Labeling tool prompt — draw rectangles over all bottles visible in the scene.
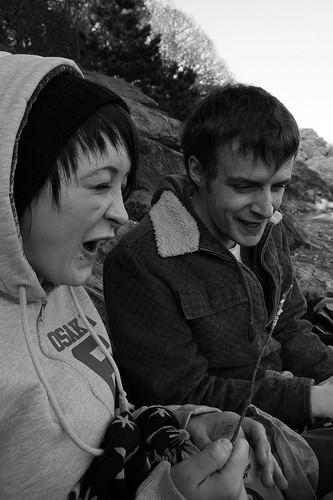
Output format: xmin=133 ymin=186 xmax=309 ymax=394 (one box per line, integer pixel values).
xmin=209 ymin=422 xmax=286 ymax=500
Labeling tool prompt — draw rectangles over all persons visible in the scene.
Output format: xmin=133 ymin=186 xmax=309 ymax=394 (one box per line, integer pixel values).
xmin=103 ymin=84 xmax=333 ymax=500
xmin=0 ymin=53 xmax=286 ymax=500
